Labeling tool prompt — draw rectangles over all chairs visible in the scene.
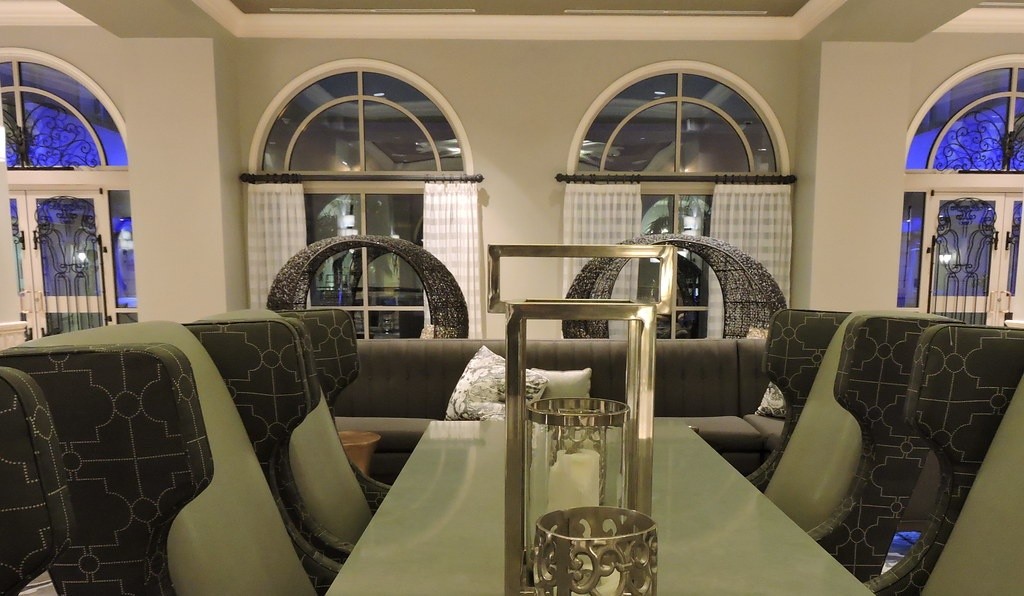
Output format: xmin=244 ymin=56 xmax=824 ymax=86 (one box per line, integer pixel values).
xmin=0 ymin=318 xmax=344 ymax=596
xmin=180 ymin=307 xmax=394 ymax=562
xmin=0 ymin=367 xmax=75 ymax=596
xmin=739 ymin=339 xmax=787 ymax=467
xmin=862 ymin=325 xmax=1024 ymax=596
xmin=745 ymin=308 xmax=966 ymax=583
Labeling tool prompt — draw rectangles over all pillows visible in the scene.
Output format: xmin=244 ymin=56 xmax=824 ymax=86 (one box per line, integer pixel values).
xmin=442 ymin=345 xmax=550 ymax=420
xmin=755 ymin=381 xmax=786 ymax=420
xmin=530 ymin=368 xmax=592 ymax=398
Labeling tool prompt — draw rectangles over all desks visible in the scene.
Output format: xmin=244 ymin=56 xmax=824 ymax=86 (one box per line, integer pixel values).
xmin=324 ymin=421 xmax=877 ymax=596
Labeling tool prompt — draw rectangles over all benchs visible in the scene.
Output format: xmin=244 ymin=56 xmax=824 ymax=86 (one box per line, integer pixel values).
xmin=332 ymin=339 xmax=763 ymax=471
